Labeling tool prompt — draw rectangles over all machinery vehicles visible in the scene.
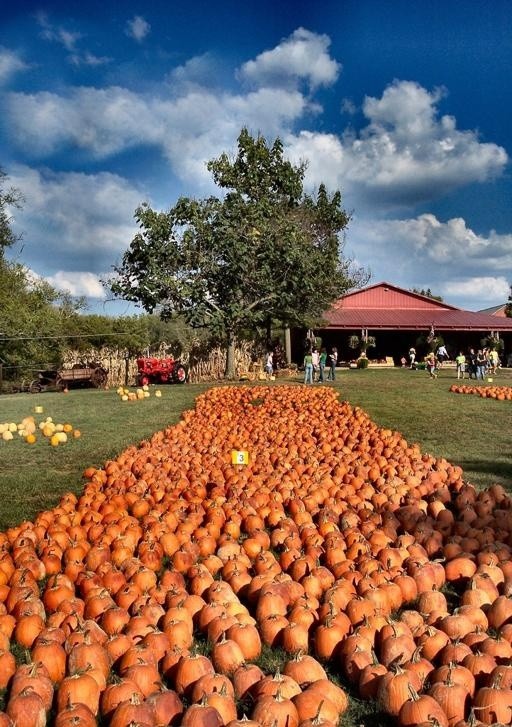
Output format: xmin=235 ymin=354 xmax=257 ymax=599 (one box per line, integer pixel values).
xmin=131 ymin=354 xmax=192 ymax=385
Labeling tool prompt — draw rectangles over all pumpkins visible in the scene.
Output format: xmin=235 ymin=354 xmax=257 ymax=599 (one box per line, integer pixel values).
xmin=0 ymin=384 xmax=512 ymax=727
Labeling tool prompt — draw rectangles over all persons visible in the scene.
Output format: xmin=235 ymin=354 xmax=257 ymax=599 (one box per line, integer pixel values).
xmin=357 ymin=352 xmax=369 ymax=361
xmin=265 ymin=351 xmax=274 ymax=376
xmin=63 ymin=354 xmax=90 ymax=369
xmin=298 ymin=347 xmax=338 ymax=385
xmin=400 ymin=333 xmax=505 ymax=382
